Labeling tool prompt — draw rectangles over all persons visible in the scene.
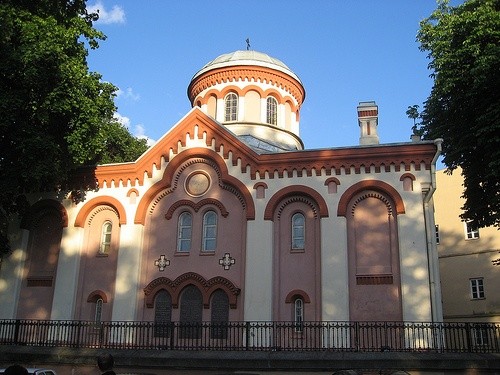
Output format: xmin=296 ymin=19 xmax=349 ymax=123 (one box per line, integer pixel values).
xmin=98 ymin=352 xmax=116 ymax=375
xmin=4 ymin=365 xmax=28 ymax=375
